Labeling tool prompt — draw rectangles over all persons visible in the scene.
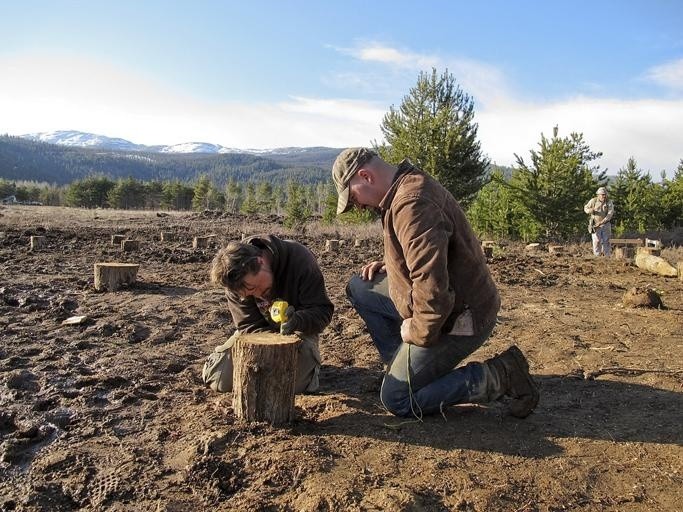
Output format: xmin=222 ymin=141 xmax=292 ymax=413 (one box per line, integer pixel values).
xmin=582 ymin=186 xmax=614 ymax=257
xmin=200 ymin=235 xmax=333 ymax=399
xmin=331 ymin=148 xmax=538 ymax=417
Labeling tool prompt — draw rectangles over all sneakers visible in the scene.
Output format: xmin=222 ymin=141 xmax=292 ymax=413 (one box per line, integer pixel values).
xmin=493 ymin=345 xmax=540 ymax=419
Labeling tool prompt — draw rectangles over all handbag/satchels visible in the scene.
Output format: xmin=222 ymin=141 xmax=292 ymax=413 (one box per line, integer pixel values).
xmin=587 ymin=218 xmax=597 ymax=234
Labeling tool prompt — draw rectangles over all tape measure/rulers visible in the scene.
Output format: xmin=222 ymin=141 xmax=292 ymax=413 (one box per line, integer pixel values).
xmin=269 ymin=300 xmax=288 ymax=336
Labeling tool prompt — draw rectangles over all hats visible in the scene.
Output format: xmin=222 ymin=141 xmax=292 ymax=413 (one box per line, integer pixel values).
xmin=596 ymin=187 xmax=608 ymax=195
xmin=331 ymin=145 xmax=379 ymax=216
